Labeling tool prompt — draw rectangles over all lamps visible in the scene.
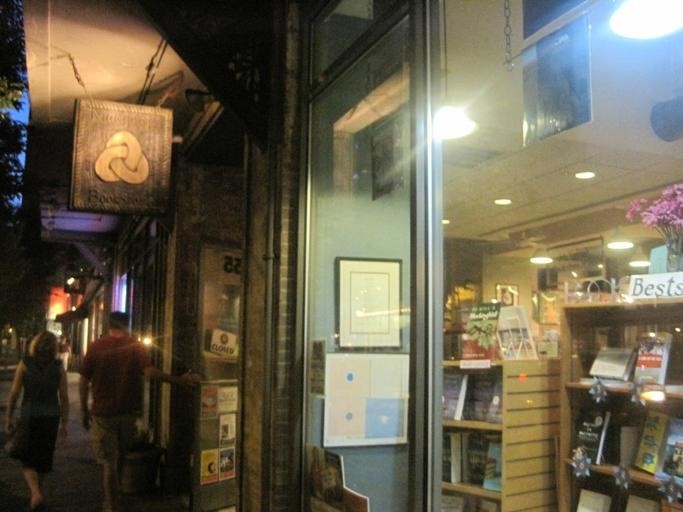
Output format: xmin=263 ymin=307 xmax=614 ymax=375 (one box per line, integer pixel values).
xmin=182 ymin=86 xmax=216 ymax=115
xmin=628 ymin=244 xmax=652 ymax=269
xmin=607 ymin=229 xmax=636 ymax=251
xmin=528 ymin=244 xmax=554 ymax=265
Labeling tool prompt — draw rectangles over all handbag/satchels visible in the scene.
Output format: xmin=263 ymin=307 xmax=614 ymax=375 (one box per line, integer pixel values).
xmin=2 ymin=422 xmax=32 ymax=460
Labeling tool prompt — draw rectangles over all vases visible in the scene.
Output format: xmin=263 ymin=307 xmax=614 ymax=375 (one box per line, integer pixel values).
xmin=663 ymin=239 xmax=682 ymax=274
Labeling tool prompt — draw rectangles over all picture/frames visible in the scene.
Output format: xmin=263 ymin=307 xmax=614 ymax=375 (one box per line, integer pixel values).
xmin=494 ymin=281 xmax=519 ymax=309
xmin=333 ymin=255 xmax=403 ymax=352
xmin=319 ymin=354 xmax=411 ymax=447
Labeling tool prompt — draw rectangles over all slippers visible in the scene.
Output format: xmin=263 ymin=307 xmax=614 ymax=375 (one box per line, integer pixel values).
xmin=20 ymin=496 xmax=46 ymax=512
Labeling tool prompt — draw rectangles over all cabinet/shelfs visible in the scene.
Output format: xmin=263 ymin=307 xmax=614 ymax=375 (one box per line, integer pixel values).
xmin=442 ymin=357 xmax=557 ymax=509
xmin=557 ymin=296 xmax=683 ymax=509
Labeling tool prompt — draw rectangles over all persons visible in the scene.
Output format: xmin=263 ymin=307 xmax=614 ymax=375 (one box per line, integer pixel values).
xmin=3 ymin=328 xmax=71 ymax=510
xmin=77 ymin=309 xmax=202 ymax=511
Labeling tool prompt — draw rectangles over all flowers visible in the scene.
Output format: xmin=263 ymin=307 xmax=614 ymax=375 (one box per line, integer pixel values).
xmin=623 ymin=176 xmax=683 ymax=240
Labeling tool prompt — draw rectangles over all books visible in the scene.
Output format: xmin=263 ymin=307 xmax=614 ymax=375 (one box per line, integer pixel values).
xmin=442 ymin=372 xmax=503 ymax=424
xmin=576 ymin=489 xmax=683 ymax=512
xmin=571 ymin=406 xmax=683 ymax=480
xmin=443 ymin=301 xmax=537 ymax=360
xmin=440 ymin=491 xmax=499 ymax=512
xmin=442 ymin=428 xmax=502 ymax=493
xmin=588 ymin=331 xmax=673 ymax=385
